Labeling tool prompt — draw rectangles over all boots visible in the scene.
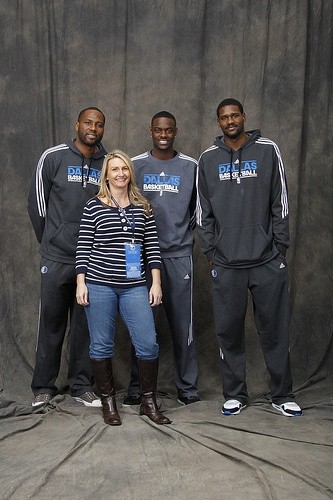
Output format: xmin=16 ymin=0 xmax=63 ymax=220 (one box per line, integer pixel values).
xmin=90 ymin=358 xmax=122 ymax=425
xmin=135 ymin=354 xmax=171 ymax=423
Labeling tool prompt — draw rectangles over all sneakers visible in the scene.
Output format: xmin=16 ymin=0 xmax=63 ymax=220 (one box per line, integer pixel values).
xmin=272 ymin=400 xmax=303 ymax=417
xmin=71 ymin=391 xmax=102 ymax=408
xmin=221 ymin=399 xmax=247 ymax=416
xmin=121 ymin=393 xmax=143 ymax=408
xmin=176 ymin=394 xmax=201 ymax=407
xmin=31 ymin=393 xmax=52 ymax=406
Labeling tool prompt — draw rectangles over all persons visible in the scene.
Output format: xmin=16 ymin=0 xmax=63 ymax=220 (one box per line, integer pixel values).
xmin=27 ymin=108 xmax=108 ymax=407
xmin=74 ymin=150 xmax=171 ymax=425
xmin=122 ymin=111 xmax=198 ymax=407
xmin=195 ymin=98 xmax=303 ymax=417
